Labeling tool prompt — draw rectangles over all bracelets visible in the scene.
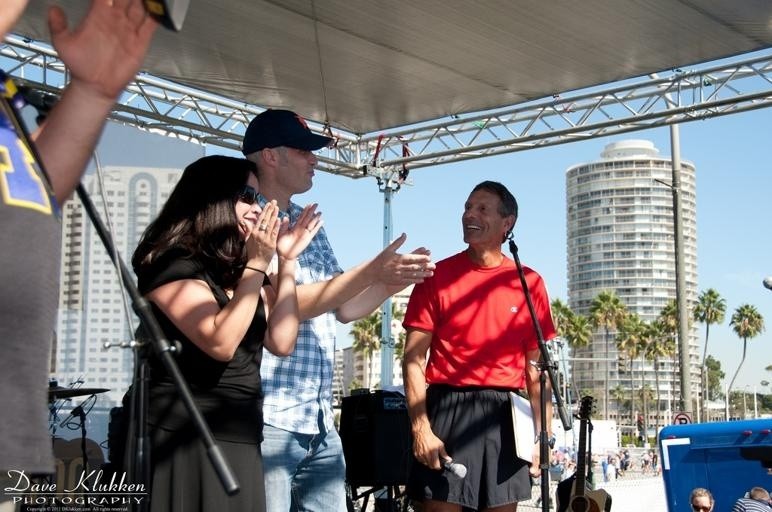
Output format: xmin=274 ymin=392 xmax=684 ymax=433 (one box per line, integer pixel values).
xmin=245 ymin=266 xmax=266 ymax=276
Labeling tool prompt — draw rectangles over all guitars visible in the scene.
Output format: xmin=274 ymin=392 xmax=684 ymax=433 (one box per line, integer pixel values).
xmin=555 ymin=395 xmax=612 ymax=512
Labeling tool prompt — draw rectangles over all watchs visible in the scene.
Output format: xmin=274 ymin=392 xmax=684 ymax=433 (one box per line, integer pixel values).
xmin=534 ymin=433 xmax=556 ymax=449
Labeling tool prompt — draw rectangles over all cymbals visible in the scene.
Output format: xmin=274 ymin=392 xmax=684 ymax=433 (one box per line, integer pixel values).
xmin=48 ymin=385 xmax=110 ymax=403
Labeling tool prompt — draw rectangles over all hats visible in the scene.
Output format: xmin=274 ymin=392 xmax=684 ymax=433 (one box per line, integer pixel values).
xmin=241 ymin=108 xmax=332 ymax=156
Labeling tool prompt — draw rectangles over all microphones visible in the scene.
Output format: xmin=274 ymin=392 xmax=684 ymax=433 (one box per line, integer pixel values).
xmin=762 ymin=277 xmax=772 ymax=291
xmin=438 ymin=456 xmax=468 ymax=480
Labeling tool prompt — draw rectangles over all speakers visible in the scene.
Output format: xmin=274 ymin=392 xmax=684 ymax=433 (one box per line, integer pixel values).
xmin=340 ymin=409 xmax=409 ymax=484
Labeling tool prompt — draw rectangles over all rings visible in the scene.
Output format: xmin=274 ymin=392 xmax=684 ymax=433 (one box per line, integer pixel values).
xmin=260 ymin=224 xmax=266 ymax=230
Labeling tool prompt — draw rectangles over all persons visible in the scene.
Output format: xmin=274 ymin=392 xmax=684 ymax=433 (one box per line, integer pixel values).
xmin=534 ymin=448 xmax=658 ymax=508
xmin=402 ymin=181 xmax=557 ymax=512
xmin=123 ymin=154 xmax=324 ymax=512
xmin=690 ymin=488 xmax=715 ymax=512
xmin=242 ymin=110 xmax=436 ymax=512
xmin=0 ymin=0 xmax=162 ymax=512
xmin=732 ymin=487 xmax=772 ymax=512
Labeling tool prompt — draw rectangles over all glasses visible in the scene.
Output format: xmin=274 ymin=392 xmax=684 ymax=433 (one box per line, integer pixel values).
xmin=239 ymin=186 xmax=260 ymax=205
